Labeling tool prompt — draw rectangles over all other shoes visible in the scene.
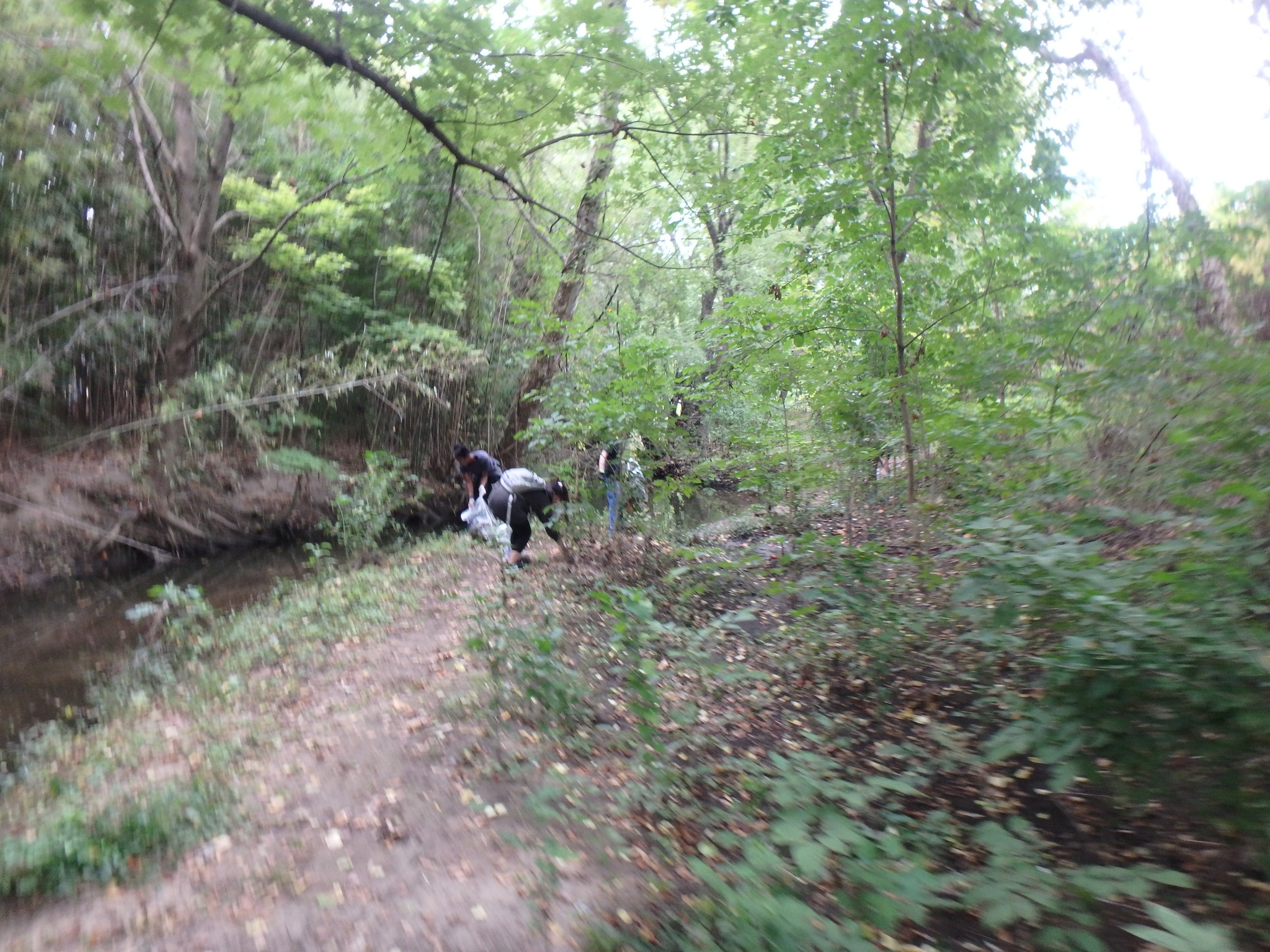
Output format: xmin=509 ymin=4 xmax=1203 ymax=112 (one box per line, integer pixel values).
xmin=505 ymin=557 xmax=530 ymax=568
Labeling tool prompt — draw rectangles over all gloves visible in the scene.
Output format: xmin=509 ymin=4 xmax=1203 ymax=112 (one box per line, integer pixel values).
xmin=478 ymin=484 xmax=486 ymax=494
xmin=598 ymin=473 xmax=606 ymax=482
xmin=468 ymin=498 xmax=475 ymax=509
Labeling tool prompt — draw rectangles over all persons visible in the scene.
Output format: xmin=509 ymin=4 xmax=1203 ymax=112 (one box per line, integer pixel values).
xmin=598 ymin=430 xmax=646 ymax=537
xmin=454 ymin=443 xmax=504 ymax=506
xmin=488 ymin=468 xmax=570 ymax=568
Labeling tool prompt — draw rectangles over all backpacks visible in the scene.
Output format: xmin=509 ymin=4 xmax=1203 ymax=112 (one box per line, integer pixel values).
xmin=499 ymin=467 xmax=548 ymax=497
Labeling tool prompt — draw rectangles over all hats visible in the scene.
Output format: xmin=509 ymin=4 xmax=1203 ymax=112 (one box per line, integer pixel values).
xmin=555 ymin=481 xmax=570 ymax=502
